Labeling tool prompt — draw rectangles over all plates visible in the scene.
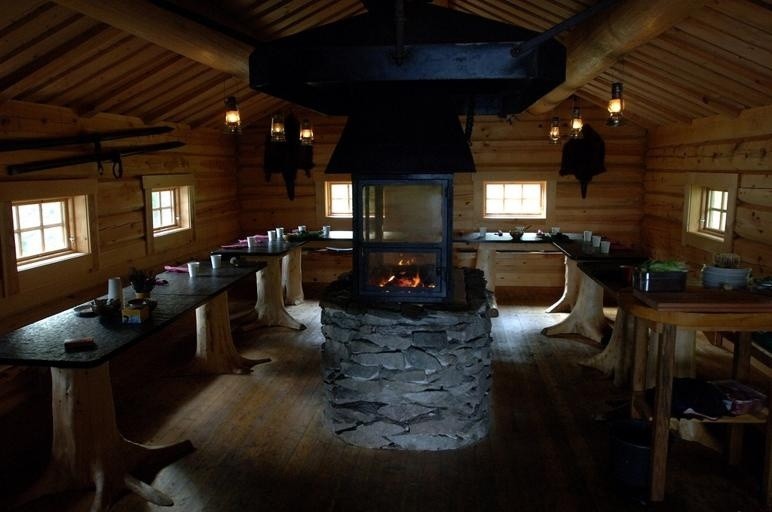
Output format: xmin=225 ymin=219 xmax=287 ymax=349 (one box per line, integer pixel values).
xmin=73 ymin=304 xmax=96 ymax=314
xmin=700 ymin=263 xmax=749 ymax=290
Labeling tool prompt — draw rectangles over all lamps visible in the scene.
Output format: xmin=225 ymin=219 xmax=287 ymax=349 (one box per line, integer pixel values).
xmin=270 ymin=109 xmax=285 ymax=142
xmin=549 ymin=106 xmax=561 ymax=143
xmin=570 ymin=94 xmax=583 ymax=139
xmin=224 ymin=76 xmax=242 ymax=136
xmin=606 ymin=58 xmax=625 ymax=125
xmin=299 ymin=118 xmax=314 ymax=145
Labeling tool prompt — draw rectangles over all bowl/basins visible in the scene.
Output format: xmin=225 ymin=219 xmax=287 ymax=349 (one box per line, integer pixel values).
xmin=509 ymin=230 xmax=524 ymax=238
xmin=282 ymin=233 xmax=298 ymax=243
xmin=127 ymin=298 xmax=158 ymax=312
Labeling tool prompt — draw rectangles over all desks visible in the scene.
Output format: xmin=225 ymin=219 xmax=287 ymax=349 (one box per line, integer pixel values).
xmin=550 ymin=231 xmax=772 ymax=512
xmin=2 ymin=232 xmax=325 ymax=509
xmin=308 ymin=230 xmax=566 ymax=287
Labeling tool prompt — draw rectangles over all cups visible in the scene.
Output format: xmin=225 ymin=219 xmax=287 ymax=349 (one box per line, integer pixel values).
xmin=479 ymin=227 xmax=487 ymax=238
xmin=552 ymin=226 xmax=560 ymax=236
xmin=592 ymin=236 xmax=601 ymax=248
xmin=298 ymin=225 xmax=306 ymax=232
xmin=267 ymin=230 xmax=276 ymax=242
xmin=186 ymin=262 xmax=200 ymax=278
xmin=583 ymin=230 xmax=592 ymax=242
xmin=323 ymin=225 xmax=331 ymax=236
xmin=247 ymin=236 xmax=256 ymax=248
xmin=276 ymin=227 xmax=285 ymax=239
xmin=209 ymin=254 xmax=222 ymax=270
xmin=107 ymin=276 xmax=126 ymax=312
xmin=600 ymin=240 xmax=611 ymax=254
